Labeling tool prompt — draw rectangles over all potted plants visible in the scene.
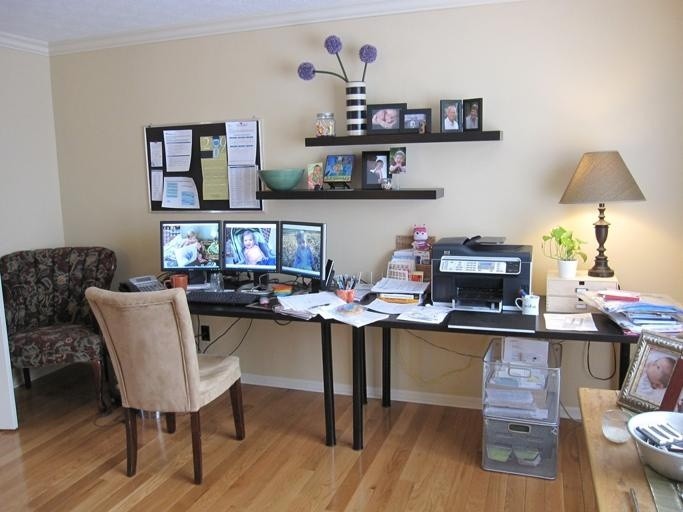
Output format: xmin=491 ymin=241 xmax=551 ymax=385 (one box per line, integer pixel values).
xmin=540 ymin=228 xmax=587 ymax=277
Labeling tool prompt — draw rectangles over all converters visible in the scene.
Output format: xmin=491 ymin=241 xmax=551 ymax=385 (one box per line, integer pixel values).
xmin=360 ymin=293 xmax=377 ymax=305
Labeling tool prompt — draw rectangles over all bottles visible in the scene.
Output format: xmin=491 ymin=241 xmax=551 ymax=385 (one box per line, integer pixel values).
xmin=314 ymin=112 xmax=337 ymax=137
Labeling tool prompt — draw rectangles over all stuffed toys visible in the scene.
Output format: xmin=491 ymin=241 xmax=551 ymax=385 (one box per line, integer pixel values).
xmin=411 ymin=224 xmax=431 ymax=251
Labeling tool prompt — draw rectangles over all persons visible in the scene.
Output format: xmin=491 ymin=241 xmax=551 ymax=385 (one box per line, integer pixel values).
xmin=367 ymin=155 xmax=384 ymax=184
xmin=389 ymin=150 xmax=406 ymax=174
xmin=372 ymin=109 xmax=400 ymax=129
xmin=181 ymin=230 xmax=208 ymax=263
xmin=308 ymin=165 xmax=323 ymax=189
xmin=242 ymin=231 xmax=267 ymax=265
xmin=294 ymin=230 xmax=315 ymax=271
xmin=445 ymin=105 xmax=459 ymax=130
xmin=635 ymin=357 xmax=676 ymax=407
xmin=327 ymin=164 xmax=346 ymax=176
xmin=466 ymin=104 xmax=479 ymax=129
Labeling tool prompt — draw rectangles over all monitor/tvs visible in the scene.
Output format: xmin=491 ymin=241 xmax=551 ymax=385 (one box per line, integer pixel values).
xmin=279 ymin=221 xmax=327 ymax=296
xmin=223 ymin=220 xmax=279 ymax=294
xmin=160 ymin=220 xmax=223 ymax=284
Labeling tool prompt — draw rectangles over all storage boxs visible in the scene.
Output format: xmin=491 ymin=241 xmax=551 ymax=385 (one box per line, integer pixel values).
xmin=479 ymin=338 xmax=562 ymax=480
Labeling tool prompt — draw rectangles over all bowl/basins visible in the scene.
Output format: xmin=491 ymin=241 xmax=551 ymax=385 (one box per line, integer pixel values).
xmin=627 ymin=410 xmax=683 ymax=483
xmin=256 ymin=168 xmax=305 ymax=191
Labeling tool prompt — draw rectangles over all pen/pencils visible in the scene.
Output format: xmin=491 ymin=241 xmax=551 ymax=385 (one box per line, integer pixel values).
xmin=380 ymin=297 xmax=417 ymax=303
xmin=309 ymin=303 xmax=330 ymax=310
xmin=519 ymin=289 xmax=530 ymax=299
xmin=338 ymin=272 xmax=361 ymax=289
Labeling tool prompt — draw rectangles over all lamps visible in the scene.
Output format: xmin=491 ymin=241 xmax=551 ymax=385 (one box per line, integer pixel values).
xmin=558 ymin=152 xmax=646 ymax=277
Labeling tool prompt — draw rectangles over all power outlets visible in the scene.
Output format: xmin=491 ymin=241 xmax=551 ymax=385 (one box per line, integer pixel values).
xmin=201 ymin=325 xmax=210 ymax=342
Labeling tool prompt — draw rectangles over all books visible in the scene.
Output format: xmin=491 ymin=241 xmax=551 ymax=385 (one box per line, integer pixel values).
xmin=448 ymin=310 xmax=536 ymax=334
xmin=622 ymin=311 xmax=683 ymax=336
xmin=377 ymin=292 xmax=426 ymax=304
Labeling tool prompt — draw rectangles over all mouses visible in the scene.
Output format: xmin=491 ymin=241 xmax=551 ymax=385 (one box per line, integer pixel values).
xmin=259 ymin=297 xmax=269 ymax=304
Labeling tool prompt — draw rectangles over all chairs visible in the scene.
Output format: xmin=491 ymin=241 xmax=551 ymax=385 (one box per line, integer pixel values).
xmin=0 ymin=246 xmax=117 ymax=414
xmin=83 ymin=286 xmax=246 ymax=482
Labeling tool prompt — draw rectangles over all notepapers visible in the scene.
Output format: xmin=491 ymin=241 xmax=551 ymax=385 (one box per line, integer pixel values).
xmin=272 ymin=285 xmax=292 ymax=296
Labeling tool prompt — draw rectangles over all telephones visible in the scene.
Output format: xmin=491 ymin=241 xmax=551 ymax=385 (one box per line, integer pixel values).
xmin=119 ymin=275 xmax=165 ymax=292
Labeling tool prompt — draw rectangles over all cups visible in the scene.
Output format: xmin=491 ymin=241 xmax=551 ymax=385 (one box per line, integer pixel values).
xmin=514 ymin=295 xmax=539 ymax=315
xmin=602 ymin=409 xmax=635 ymax=444
xmin=210 ymin=273 xmax=224 ymax=291
xmin=335 ymin=288 xmax=355 ymax=304
xmin=164 ymin=274 xmax=188 ymax=293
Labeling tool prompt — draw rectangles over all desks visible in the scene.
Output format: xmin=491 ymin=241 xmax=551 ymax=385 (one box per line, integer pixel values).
xmin=578 ymin=386 xmax=683 ymax=512
xmin=187 ymin=274 xmax=640 ymax=450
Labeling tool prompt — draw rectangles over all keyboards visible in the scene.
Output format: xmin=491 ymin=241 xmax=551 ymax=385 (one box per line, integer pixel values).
xmin=187 ymin=291 xmax=260 ymax=306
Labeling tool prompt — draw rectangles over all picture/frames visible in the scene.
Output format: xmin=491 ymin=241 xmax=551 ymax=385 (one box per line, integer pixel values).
xmin=399 ymin=108 xmax=431 ymax=133
xmin=366 ymin=103 xmax=407 ymax=133
xmin=617 ymin=329 xmax=682 ymax=416
xmin=323 ymin=154 xmax=354 ymax=185
xmin=463 ymin=98 xmax=483 ymax=133
xmin=361 ymin=150 xmax=389 ymax=190
xmin=440 ymin=99 xmax=463 ymax=134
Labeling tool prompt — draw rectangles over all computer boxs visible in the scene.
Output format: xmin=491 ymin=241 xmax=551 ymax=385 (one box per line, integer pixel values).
xmin=102 ymin=343 xmax=122 ymax=408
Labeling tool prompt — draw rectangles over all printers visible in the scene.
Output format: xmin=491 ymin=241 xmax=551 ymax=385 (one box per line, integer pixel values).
xmin=430 ymin=235 xmax=534 ymax=314
